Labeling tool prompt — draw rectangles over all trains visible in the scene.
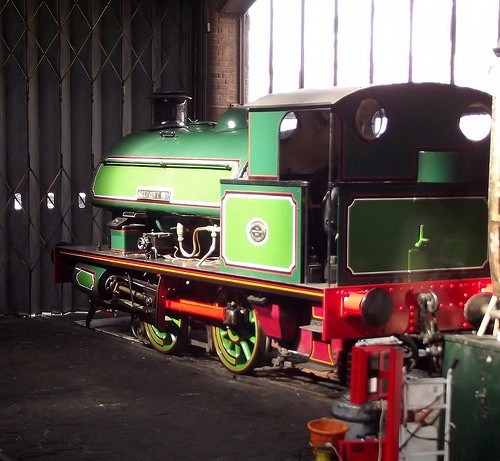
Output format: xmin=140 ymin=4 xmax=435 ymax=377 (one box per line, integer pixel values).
xmin=57 ymin=80 xmax=500 ymax=395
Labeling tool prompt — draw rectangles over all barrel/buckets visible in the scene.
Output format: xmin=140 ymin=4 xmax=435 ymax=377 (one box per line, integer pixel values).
xmin=307 ymin=418 xmax=350 ymax=457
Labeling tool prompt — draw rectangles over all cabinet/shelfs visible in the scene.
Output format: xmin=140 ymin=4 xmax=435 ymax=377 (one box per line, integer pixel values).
xmin=444 ymin=334 xmax=500 ymax=461
